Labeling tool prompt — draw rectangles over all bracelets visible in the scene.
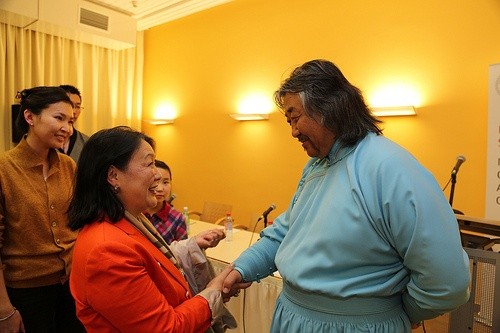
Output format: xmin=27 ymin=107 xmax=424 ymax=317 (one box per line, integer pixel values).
xmin=0 ymin=307 xmax=16 ymax=321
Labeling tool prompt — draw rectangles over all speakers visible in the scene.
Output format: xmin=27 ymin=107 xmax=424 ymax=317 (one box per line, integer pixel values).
xmin=451 ymin=155 xmax=465 ymax=175
xmin=259 ymin=204 xmax=277 ymax=220
xmin=11 ymin=104 xmax=27 ymax=142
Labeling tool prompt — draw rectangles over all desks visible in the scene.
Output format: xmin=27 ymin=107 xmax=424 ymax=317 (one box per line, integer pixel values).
xmin=189 ymin=219 xmax=283 ymax=333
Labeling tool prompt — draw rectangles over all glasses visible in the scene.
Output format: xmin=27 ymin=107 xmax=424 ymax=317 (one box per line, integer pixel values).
xmin=72 ymin=105 xmax=87 ymax=114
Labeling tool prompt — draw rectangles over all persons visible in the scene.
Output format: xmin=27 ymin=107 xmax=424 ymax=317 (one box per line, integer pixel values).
xmin=59 ymin=86 xmax=90 ymax=163
xmin=0 ymin=86 xmax=86 ymax=333
xmin=207 ymin=59 xmax=471 ymax=333
xmin=144 ymin=160 xmax=189 ymax=246
xmin=65 ymin=126 xmax=253 ymax=333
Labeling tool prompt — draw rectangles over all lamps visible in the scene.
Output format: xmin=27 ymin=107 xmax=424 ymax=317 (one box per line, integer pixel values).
xmin=370 ymin=106 xmax=418 ymax=119
xmin=230 ymin=113 xmax=270 ymax=121
xmin=145 ymin=118 xmax=174 ymax=125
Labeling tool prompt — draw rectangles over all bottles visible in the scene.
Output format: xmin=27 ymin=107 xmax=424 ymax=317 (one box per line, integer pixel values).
xmin=268 ymin=220 xmax=273 ymax=226
xmin=224 ymin=212 xmax=233 ymax=241
xmin=183 ymin=207 xmax=190 ymax=235
